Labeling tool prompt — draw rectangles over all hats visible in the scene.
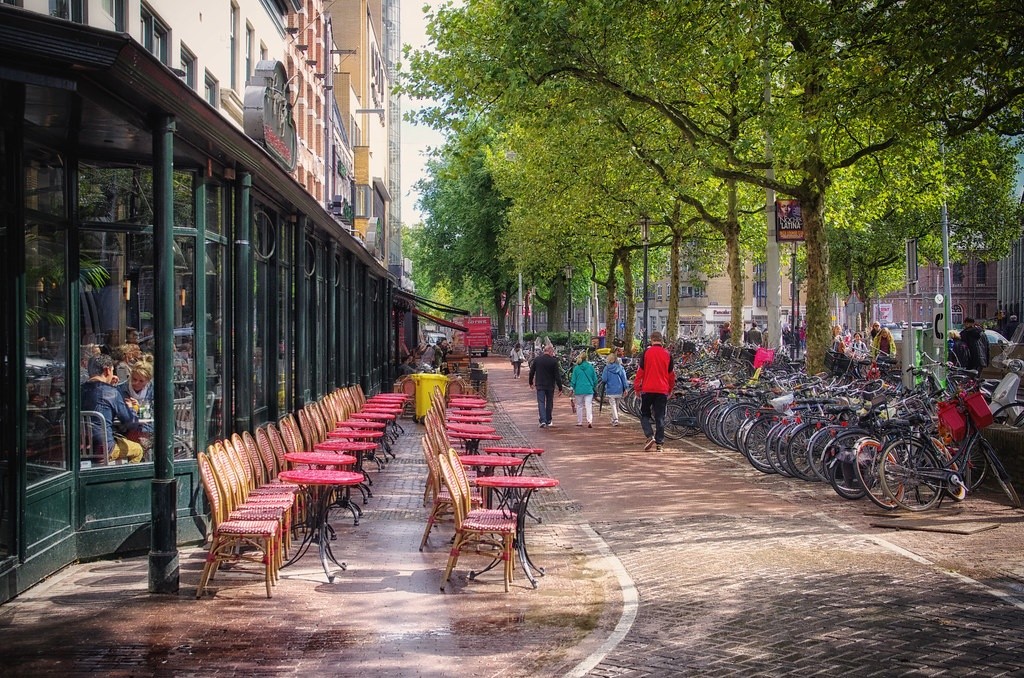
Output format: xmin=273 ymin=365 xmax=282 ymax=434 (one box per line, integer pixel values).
xmin=963 ymin=316 xmax=975 ymax=324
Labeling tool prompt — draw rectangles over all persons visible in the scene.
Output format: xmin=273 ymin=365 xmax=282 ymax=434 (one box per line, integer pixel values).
xmin=947 ymin=316 xmax=990 ymax=377
xmin=761 ymin=323 xmax=768 ymax=334
xmin=80 ymin=343 xmax=154 ymax=464
xmin=1002 ymin=315 xmax=1020 ymax=341
xmin=782 ymin=325 xmax=806 ymax=349
xmin=602 ymin=353 xmax=628 ymax=426
xmin=720 ymin=321 xmax=730 ymax=342
xmin=569 ymin=352 xmax=598 ymax=429
xmin=744 ymin=323 xmax=747 ymax=342
xmin=831 ymin=321 xmax=896 ymax=361
xmin=399 ymin=337 xmax=453 ymax=376
xmin=634 ymin=331 xmax=675 ymax=452
xmin=748 ymin=322 xmax=763 ymax=346
xmin=529 ymin=345 xmax=562 ymax=428
xmin=509 ymin=342 xmax=527 ymax=378
xmin=510 ymin=328 xmax=515 ymax=334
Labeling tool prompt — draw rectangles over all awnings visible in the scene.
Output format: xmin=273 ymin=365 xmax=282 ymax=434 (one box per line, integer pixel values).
xmin=395 ymin=287 xmax=470 ymax=315
xmin=413 ymin=307 xmax=469 ymax=332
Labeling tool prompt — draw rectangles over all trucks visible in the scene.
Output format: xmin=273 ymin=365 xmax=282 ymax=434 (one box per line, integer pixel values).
xmin=447 ymin=316 xmax=492 ymax=357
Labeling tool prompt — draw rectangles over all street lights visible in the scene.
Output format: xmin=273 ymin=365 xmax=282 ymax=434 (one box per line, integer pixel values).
xmin=532 ymin=285 xmax=536 ymax=334
xmin=640 ymin=217 xmax=649 ymax=349
xmin=564 ymin=261 xmax=573 ymax=337
xmin=794 ymin=279 xmax=803 ymax=357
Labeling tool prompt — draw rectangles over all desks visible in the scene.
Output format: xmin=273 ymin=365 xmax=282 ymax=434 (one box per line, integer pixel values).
xmin=277 ymin=391 xmax=559 ymax=589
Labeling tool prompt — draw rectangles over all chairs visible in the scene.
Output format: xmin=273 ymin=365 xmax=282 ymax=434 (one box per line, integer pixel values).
xmin=197 ymin=376 xmax=518 ymax=594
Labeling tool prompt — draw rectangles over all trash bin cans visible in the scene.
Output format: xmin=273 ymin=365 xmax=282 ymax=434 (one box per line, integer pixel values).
xmin=411 ymin=374 xmax=449 ymax=426
xmin=596 ymin=347 xmax=611 ymax=355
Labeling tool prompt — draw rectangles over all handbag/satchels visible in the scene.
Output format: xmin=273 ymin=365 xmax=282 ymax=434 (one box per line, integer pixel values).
xmin=519 ymin=359 xmax=523 ymax=363
xmin=965 ymin=391 xmax=994 ymax=428
xmin=593 ymin=387 xmax=598 ymax=397
xmin=935 ymin=402 xmax=965 ymax=441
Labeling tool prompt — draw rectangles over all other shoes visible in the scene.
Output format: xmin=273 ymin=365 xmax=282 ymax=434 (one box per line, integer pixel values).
xmin=588 ymin=422 xmax=592 ymax=428
xmin=514 ymin=374 xmax=517 ymax=379
xmin=518 ymin=376 xmax=520 ymax=378
xmin=656 ymin=444 xmax=663 ymax=451
xmin=644 ymin=435 xmax=656 ymax=450
xmin=576 ymin=423 xmax=582 ymax=426
xmin=540 ymin=422 xmax=546 ymax=428
xmin=611 ymin=419 xmax=619 ymax=426
xmin=548 ymin=421 xmax=553 ymax=426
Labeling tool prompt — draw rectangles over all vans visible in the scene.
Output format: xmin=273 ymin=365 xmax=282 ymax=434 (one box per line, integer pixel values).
xmin=426 ymin=331 xmax=446 ymax=347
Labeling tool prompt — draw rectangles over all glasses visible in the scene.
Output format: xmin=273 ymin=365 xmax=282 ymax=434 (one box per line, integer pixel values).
xmin=874 ymin=325 xmax=880 ymax=328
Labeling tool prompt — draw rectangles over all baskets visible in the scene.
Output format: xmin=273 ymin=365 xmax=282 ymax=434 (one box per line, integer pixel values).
xmin=824 ymin=350 xmax=851 ymax=372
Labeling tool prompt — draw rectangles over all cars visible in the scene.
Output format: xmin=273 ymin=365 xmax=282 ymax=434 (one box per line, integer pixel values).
xmin=879 ymin=321 xmax=933 ymax=330
xmin=984 ymin=330 xmax=1009 ymax=344
xmin=137 ymin=328 xmax=194 ymax=353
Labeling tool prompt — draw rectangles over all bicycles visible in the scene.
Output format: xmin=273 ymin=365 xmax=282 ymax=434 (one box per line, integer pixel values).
xmin=491 ymin=334 xmax=1024 ymax=512
xmin=25 ymin=358 xmax=192 ymax=461
